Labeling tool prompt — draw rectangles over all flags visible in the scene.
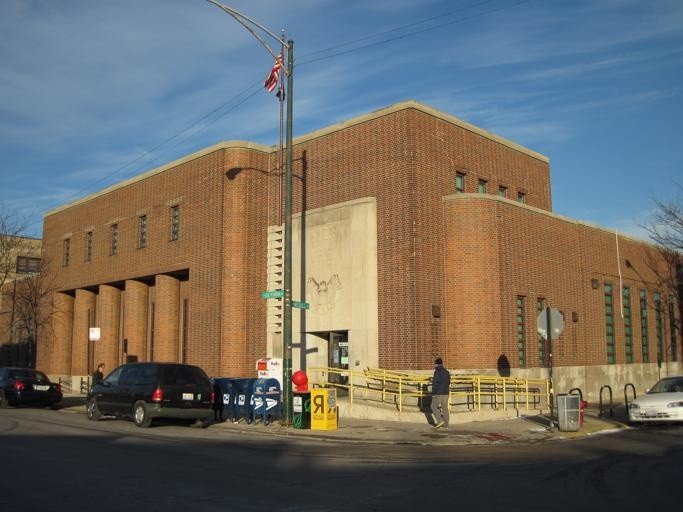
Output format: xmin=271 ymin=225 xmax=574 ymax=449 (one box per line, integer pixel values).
xmin=263 ymin=50 xmax=282 ymax=94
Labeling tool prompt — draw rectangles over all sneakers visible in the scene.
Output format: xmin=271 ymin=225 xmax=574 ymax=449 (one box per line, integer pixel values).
xmin=435 ymin=421 xmax=444 ymax=428
xmin=437 ymin=425 xmax=448 ymax=429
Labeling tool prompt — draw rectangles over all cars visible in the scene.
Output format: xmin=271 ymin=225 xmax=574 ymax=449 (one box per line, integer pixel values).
xmin=0 ymin=366 xmax=64 ymax=409
xmin=627 ymin=375 xmax=683 ymax=425
xmin=84 ymin=361 xmax=216 ymax=429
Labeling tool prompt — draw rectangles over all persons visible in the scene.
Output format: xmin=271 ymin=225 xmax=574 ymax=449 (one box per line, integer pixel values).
xmin=428 ymin=358 xmax=449 ymax=429
xmin=90 ymin=362 xmax=105 ymax=388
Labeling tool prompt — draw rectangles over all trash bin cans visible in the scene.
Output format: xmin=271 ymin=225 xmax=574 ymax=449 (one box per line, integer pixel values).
xmin=210 ymin=378 xmax=281 ymax=426
xmin=556 ymin=394 xmax=580 ymax=432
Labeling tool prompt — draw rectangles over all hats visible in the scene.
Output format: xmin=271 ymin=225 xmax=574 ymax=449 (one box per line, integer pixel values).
xmin=434 ymin=358 xmax=442 ymax=364
